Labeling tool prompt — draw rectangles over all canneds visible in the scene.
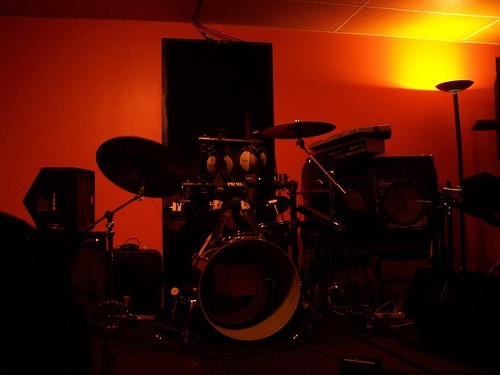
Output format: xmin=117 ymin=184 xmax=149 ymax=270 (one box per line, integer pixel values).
xmin=123 ymin=295 xmax=132 ymax=306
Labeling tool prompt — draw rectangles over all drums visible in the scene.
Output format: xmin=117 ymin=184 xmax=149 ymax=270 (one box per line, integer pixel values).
xmin=194 ymin=235 xmax=300 ymax=341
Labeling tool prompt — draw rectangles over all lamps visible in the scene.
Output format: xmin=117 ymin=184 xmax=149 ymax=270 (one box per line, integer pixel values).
xmin=436 ymin=78 xmax=475 ymax=278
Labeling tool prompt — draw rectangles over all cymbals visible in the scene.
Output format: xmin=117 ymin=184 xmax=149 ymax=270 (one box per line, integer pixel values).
xmin=96 ymin=137 xmax=190 ymax=198
xmin=262 ymin=122 xmax=336 ymax=138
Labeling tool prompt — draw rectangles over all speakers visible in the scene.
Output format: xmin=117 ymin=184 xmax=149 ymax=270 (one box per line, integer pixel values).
xmin=112 ymin=248 xmax=162 ymax=312
xmin=336 ymin=155 xmax=443 ymax=247
xmin=0 ymin=240 xmax=107 ymax=375
